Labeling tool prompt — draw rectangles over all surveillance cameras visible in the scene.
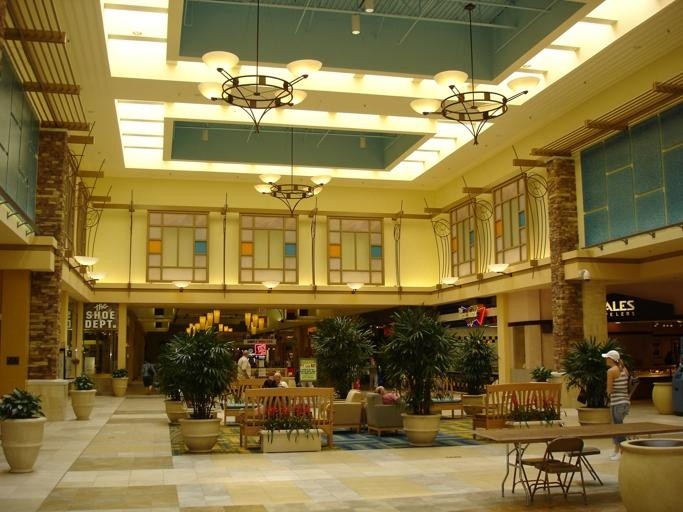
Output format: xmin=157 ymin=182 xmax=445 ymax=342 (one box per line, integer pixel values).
xmin=583 ymin=271 xmax=591 ymax=281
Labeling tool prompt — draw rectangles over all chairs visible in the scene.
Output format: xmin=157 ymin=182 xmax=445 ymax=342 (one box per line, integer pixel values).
xmin=511 ymin=442 xmax=565 ymax=495
xmin=559 ymin=447 xmax=605 ymax=487
xmin=532 ymin=437 xmax=586 ymax=508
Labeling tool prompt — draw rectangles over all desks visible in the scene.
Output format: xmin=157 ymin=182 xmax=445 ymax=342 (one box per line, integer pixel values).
xmin=472 ymin=421 xmax=683 ymax=505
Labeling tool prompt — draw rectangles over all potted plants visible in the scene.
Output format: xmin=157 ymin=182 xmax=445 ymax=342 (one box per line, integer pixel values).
xmin=383 ymin=307 xmax=462 ymax=444
xmin=558 ymin=337 xmax=630 ymax=426
xmin=0 ymin=388 xmax=46 ymax=473
xmin=111 ymin=368 xmax=130 ymax=397
xmin=171 ymin=330 xmax=238 ymax=452
xmin=157 ymin=332 xmax=193 ymax=424
xmin=451 ymin=332 xmax=499 ymax=416
xmin=69 ymin=374 xmax=97 ymax=420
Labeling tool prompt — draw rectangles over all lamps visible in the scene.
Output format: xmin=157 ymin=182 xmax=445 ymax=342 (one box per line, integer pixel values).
xmin=198 ymin=0 xmax=322 ymax=135
xmin=69 ymin=253 xmax=98 ymax=272
xmin=347 ymin=282 xmax=363 ymax=293
xmin=262 ymin=280 xmax=280 ymax=292
xmin=412 ymin=2 xmax=540 ymax=145
xmin=86 ymin=269 xmax=105 ymax=286
xmin=172 ymin=280 xmax=192 ymax=292
xmin=255 ymin=126 xmax=331 ymax=216
xmin=443 ymin=277 xmax=461 ymax=288
xmin=487 ymin=264 xmax=512 ymax=276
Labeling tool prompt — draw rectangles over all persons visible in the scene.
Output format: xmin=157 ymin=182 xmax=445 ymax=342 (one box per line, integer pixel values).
xmin=273 ymin=372 xmax=288 ymax=387
xmin=601 ymin=350 xmax=632 ymax=459
xmin=237 ymin=350 xmax=252 ymax=380
xmin=375 ymin=387 xmax=400 ymax=404
xmin=262 ymin=380 xmax=288 ymax=407
xmin=141 ymin=360 xmax=158 ymax=394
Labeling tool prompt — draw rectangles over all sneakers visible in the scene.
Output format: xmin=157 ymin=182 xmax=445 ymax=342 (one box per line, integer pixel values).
xmin=611 ymin=453 xmax=621 ymax=460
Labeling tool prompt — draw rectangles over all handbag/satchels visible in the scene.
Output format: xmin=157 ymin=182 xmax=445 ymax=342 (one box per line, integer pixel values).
xmin=629 ymin=376 xmax=639 ymax=397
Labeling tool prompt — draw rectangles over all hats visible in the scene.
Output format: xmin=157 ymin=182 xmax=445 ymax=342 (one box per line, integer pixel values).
xmin=602 ymin=350 xmax=620 ymax=362
